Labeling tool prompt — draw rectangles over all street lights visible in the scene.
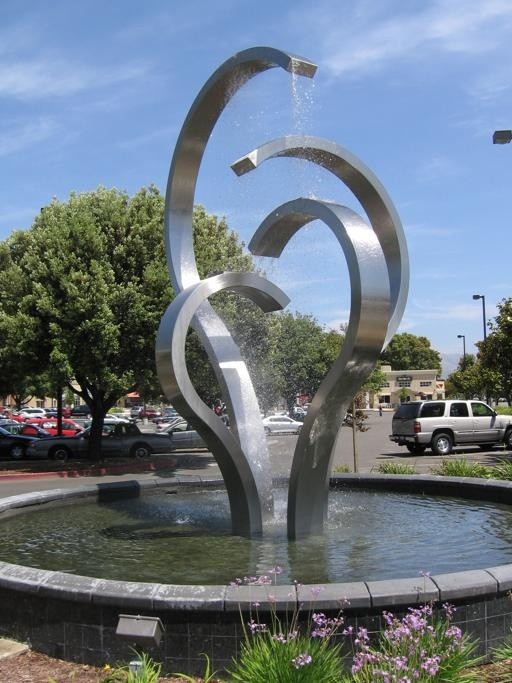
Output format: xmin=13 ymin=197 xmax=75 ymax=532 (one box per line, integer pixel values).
xmin=457 ymin=334 xmax=467 ymax=373
xmin=491 ymin=129 xmax=512 ymax=145
xmin=472 ymin=293 xmax=491 ymax=405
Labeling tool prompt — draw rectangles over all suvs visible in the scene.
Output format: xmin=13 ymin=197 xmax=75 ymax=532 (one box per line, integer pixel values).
xmin=388 ymin=398 xmax=512 ymax=454
